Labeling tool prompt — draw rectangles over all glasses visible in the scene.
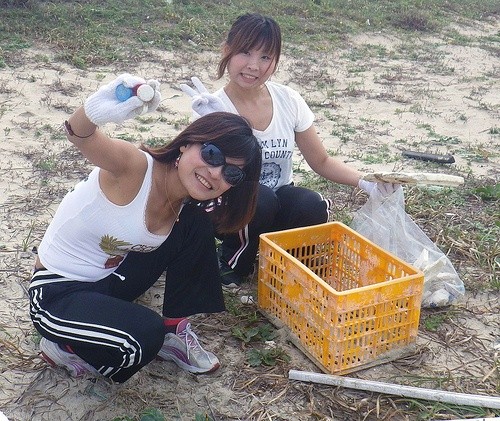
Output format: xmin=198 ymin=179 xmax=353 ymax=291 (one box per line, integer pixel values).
xmin=199 ymin=141 xmax=246 ymax=188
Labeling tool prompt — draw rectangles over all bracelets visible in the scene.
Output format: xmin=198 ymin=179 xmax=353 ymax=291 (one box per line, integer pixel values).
xmin=64 ymin=120 xmax=95 ymax=138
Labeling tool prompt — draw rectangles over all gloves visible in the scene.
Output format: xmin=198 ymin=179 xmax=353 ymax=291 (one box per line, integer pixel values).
xmin=180 ymin=77 xmax=227 ymax=116
xmin=359 ymin=173 xmax=400 ymax=196
xmin=84 ymin=73 xmax=160 ymax=126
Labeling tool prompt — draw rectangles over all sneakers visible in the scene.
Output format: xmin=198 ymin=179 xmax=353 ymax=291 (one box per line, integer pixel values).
xmin=215 ymin=253 xmax=240 ymax=288
xmin=39 ymin=337 xmax=92 ymax=376
xmin=158 ymin=319 xmax=221 ymax=374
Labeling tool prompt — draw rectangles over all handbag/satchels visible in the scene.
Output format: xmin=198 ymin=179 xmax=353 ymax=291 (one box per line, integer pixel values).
xmin=348 ymin=183 xmax=465 ymax=307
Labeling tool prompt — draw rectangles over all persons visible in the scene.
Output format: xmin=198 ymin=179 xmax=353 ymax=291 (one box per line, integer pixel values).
xmin=179 ymin=13 xmax=399 ymax=288
xmin=27 ymin=72 xmax=263 ymax=384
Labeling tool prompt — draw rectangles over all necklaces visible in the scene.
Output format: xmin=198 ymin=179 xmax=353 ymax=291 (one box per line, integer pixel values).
xmin=163 ymin=176 xmax=179 ymax=222
